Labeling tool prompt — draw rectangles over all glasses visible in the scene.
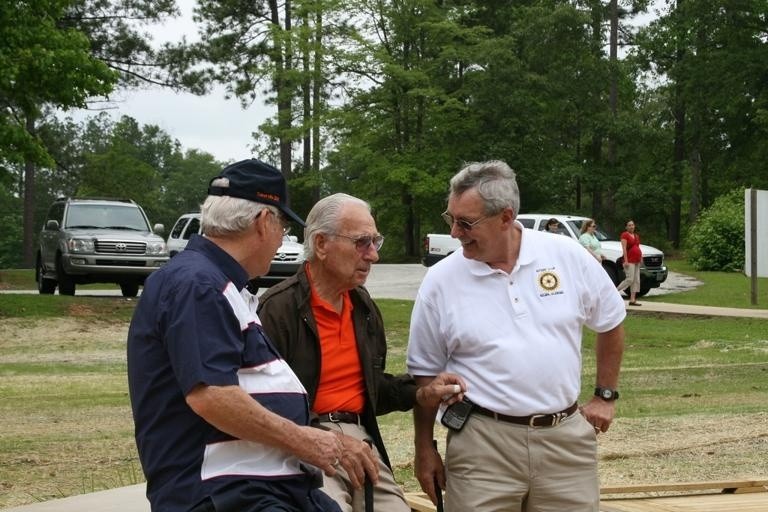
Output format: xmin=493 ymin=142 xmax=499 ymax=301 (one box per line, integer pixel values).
xmin=442 ymin=210 xmax=492 ymax=229
xmin=321 ymin=233 xmax=384 ymax=254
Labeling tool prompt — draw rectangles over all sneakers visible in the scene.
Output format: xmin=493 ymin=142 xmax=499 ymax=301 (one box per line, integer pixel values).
xmin=629 ymin=302 xmax=641 ymax=306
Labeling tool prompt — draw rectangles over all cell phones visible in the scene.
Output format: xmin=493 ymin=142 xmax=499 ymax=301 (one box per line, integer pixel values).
xmin=441 ymin=393 xmax=473 ymax=432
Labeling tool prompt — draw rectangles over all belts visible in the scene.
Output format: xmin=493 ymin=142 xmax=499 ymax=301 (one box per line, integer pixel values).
xmin=319 ymin=412 xmax=365 ymax=426
xmin=463 ymin=395 xmax=577 ymax=428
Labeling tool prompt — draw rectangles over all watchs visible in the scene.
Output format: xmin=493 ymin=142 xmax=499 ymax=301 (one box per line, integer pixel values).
xmin=597 ymin=385 xmax=619 ymax=401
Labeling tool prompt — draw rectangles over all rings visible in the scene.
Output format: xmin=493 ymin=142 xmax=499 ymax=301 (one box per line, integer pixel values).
xmin=595 ymin=425 xmax=603 ymax=431
xmin=332 ymin=455 xmax=338 ymax=467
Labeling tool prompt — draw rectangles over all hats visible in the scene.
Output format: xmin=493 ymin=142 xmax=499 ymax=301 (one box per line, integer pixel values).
xmin=208 ymin=159 xmax=307 ymax=227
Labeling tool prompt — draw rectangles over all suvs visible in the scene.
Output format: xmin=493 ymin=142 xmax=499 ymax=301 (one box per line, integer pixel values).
xmin=34 ymin=197 xmax=170 ymax=297
xmin=167 ymin=211 xmax=306 ymax=293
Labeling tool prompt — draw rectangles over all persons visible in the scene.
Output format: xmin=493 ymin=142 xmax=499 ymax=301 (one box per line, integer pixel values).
xmin=576 ymin=218 xmax=604 ymax=266
xmin=613 ymin=221 xmax=642 ymax=304
xmin=542 ymin=216 xmax=560 ymax=235
xmin=125 ymin=160 xmax=342 ymax=510
xmin=408 ymin=159 xmax=627 ymax=512
xmin=260 ymin=194 xmax=466 ymax=512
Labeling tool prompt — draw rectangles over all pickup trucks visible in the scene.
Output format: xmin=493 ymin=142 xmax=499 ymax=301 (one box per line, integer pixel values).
xmin=421 ymin=214 xmax=667 ymax=298
